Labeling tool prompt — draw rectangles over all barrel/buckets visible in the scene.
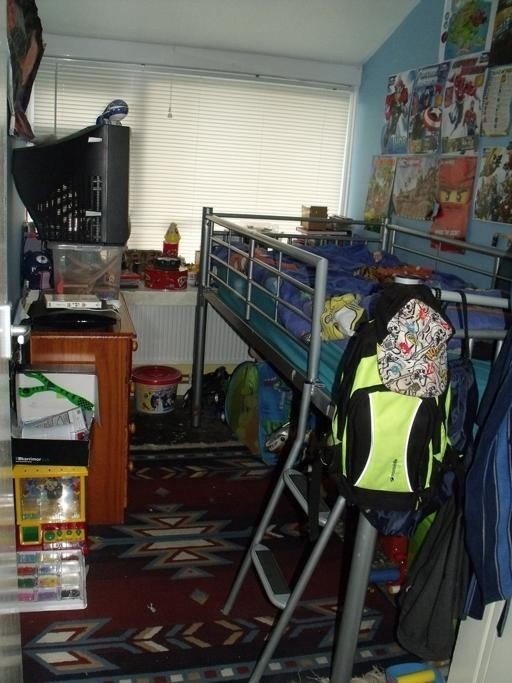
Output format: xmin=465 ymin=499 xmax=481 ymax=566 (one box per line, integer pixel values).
xmin=131 ymin=365 xmax=182 ymax=415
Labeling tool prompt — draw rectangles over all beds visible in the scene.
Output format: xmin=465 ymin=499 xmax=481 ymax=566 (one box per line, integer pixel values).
xmin=191 ymin=206 xmax=512 ymax=682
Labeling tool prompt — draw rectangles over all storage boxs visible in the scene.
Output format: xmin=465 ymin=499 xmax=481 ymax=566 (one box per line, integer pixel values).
xmin=45 ymin=239 xmax=129 ymax=301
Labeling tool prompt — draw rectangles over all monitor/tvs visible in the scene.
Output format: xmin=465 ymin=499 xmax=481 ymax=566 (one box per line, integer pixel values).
xmin=11 ymin=124 xmax=132 ymax=246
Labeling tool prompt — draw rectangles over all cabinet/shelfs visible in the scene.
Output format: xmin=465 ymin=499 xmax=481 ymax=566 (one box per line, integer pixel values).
xmin=28 ymin=292 xmax=138 ymax=528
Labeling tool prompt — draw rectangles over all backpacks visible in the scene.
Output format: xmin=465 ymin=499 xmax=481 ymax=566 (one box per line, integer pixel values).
xmin=318 ymin=281 xmax=467 ymax=511
